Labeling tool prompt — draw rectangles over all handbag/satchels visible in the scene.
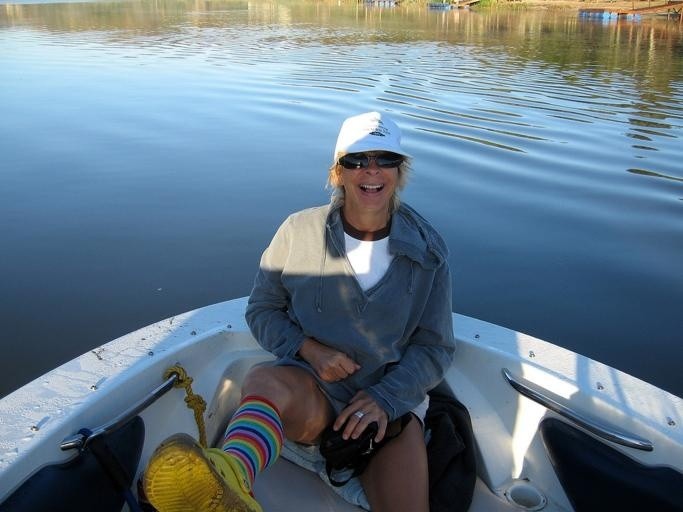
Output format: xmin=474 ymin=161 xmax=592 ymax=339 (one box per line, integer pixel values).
xmin=319 ymin=411 xmax=413 ymax=486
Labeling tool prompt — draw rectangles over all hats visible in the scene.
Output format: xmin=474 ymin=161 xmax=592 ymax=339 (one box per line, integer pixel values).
xmin=333 ymin=112 xmax=413 ymax=162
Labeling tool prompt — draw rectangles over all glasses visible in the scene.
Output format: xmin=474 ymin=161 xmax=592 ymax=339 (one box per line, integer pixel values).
xmin=337 ymin=152 xmax=405 ymax=170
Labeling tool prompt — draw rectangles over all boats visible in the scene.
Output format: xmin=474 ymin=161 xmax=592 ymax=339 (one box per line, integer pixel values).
xmin=1 ymin=296 xmax=683 ymax=512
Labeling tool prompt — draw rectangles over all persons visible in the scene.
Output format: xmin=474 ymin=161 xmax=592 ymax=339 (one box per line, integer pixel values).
xmin=143 ymin=112 xmax=456 ymax=512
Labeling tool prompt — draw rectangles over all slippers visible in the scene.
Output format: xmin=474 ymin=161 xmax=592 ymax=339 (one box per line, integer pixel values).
xmin=142 ymin=433 xmax=262 ymax=511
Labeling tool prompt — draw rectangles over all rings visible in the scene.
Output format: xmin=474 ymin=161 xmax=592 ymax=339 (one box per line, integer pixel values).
xmin=354 ymin=411 xmax=364 ymax=418
